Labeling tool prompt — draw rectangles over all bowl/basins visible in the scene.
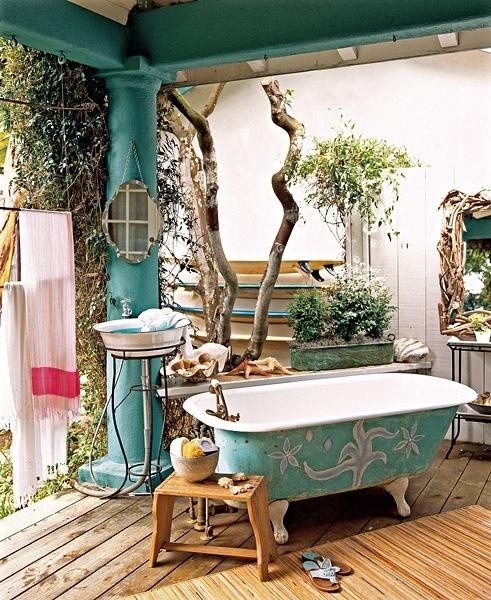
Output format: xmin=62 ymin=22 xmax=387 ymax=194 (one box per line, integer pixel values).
xmin=473 ymin=328 xmax=491 ymax=342
xmin=91 ymin=317 xmax=191 ymax=350
xmin=168 ymin=448 xmax=220 ymax=483
xmin=170 ymin=437 xmax=186 ymax=457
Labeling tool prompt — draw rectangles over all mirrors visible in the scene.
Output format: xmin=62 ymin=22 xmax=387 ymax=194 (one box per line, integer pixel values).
xmin=435 ymin=188 xmax=491 ymax=342
xmin=102 ymin=140 xmax=169 ymax=266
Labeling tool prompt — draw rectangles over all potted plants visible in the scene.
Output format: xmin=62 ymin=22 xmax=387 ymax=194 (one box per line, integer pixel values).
xmin=285 ymin=261 xmax=399 ymax=370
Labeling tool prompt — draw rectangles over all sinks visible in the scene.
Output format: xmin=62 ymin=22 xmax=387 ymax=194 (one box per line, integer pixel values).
xmin=93 ymin=318 xmax=190 ymax=333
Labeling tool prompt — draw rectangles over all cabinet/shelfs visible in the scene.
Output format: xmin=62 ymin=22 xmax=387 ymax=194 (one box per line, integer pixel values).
xmin=445 ymin=333 xmax=491 ymax=459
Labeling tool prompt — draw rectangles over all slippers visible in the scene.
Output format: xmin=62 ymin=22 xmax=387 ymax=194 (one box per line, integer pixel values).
xmin=303 ymin=561 xmax=340 ymax=592
xmin=302 ymin=552 xmax=351 ymax=576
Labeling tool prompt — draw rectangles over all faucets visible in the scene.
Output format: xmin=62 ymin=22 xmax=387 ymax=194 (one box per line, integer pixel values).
xmin=205 ymin=379 xmax=240 ymax=421
xmin=120 ymin=297 xmax=133 ymax=317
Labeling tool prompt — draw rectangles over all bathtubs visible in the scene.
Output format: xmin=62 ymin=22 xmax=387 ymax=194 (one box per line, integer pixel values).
xmin=182 ymin=372 xmax=478 ymax=507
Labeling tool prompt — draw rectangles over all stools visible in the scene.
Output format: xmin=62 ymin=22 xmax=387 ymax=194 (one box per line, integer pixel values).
xmin=149 ymin=471 xmax=278 ymax=583
